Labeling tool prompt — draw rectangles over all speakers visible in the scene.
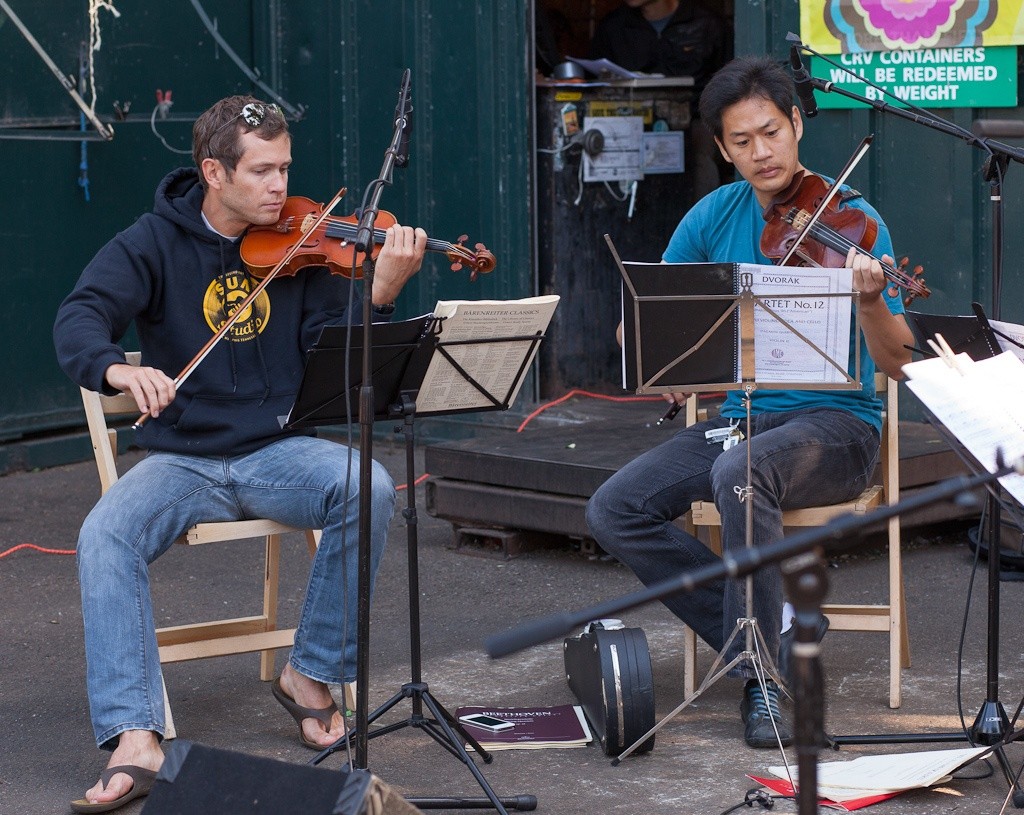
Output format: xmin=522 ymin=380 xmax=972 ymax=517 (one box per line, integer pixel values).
xmin=138 ymin=739 xmax=423 ymax=815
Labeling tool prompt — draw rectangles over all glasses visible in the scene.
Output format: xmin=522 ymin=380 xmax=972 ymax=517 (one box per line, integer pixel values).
xmin=207 ymin=103 xmax=280 ymax=156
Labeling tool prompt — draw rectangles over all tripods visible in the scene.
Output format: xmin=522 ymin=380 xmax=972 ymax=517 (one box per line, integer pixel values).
xmin=829 ymin=303 xmax=1024 ymax=808
xmin=631 ymin=287 xmax=841 ymax=803
xmin=304 ymin=314 xmax=550 ymax=815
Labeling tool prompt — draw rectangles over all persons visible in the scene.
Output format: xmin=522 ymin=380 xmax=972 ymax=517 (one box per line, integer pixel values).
xmin=50 ymin=99 xmax=429 ymax=815
xmin=543 ymin=0 xmax=917 ymax=748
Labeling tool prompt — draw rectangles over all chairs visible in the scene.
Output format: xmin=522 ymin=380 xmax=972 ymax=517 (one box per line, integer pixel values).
xmin=80 ymin=351 xmax=356 ymax=740
xmin=684 ymin=372 xmax=912 ymax=710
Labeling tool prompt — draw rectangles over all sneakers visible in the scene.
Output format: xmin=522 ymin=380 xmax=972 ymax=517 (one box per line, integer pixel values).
xmin=741 ymin=678 xmax=792 ymax=745
xmin=778 ymin=608 xmax=830 ymax=700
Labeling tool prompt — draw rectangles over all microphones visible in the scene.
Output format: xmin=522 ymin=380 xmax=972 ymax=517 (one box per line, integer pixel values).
xmin=392 ymin=72 xmax=411 ymax=168
xmin=790 ymin=47 xmax=818 ymax=118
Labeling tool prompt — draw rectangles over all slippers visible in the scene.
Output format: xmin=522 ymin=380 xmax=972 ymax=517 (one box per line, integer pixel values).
xmin=270 ymin=678 xmax=354 ymax=750
xmin=71 ymin=765 xmax=159 ymax=813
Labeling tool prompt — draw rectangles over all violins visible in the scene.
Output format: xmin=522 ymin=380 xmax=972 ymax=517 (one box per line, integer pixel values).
xmin=240 ymin=196 xmax=496 ymax=281
xmin=759 ymin=175 xmax=933 ymax=308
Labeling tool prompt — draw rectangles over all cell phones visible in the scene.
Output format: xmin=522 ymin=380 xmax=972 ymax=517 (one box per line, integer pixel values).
xmin=458 ymin=713 xmax=516 ymax=733
